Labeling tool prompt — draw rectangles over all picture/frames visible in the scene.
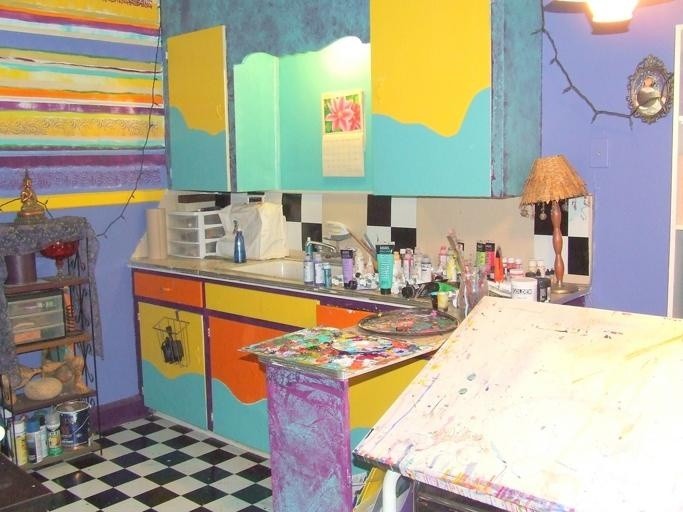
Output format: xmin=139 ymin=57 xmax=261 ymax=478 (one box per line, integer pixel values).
xmin=623 ymin=51 xmax=676 ymax=126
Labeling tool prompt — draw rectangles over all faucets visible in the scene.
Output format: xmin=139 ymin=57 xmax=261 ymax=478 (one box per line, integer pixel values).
xmin=303 ymin=240 xmax=337 ymax=258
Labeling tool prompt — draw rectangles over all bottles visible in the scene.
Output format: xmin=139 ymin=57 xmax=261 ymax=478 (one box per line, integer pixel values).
xmin=6 ymin=410 xmax=61 ymax=466
xmin=304 ymin=237 xmax=312 ymax=257
xmin=233 ymin=229 xmax=247 ymax=264
xmin=502 ymin=257 xmax=545 ymax=277
xmin=303 ymin=253 xmax=332 ymax=290
xmin=392 ymin=246 xmax=432 ymax=283
xmin=456 ymin=270 xmax=489 ymax=319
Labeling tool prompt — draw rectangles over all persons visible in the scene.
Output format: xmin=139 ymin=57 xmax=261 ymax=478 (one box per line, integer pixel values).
xmin=637 ymin=74 xmax=657 ymax=108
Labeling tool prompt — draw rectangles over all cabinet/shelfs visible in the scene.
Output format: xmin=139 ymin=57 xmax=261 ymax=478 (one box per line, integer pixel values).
xmin=132 ymin=269 xmax=210 ymax=430
xmin=159 ymin=1 xmax=279 ymax=195
xmin=166 ymin=209 xmax=226 ymax=259
xmin=5 ymin=288 xmax=66 ymax=346
xmin=1 ymin=216 xmax=102 ymax=471
xmin=368 ymin=0 xmax=542 ymax=199
xmin=315 ymin=296 xmax=432 ymax=327
xmin=204 ymin=282 xmax=319 ymax=456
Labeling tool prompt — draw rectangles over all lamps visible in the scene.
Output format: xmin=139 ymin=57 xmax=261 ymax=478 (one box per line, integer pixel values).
xmin=542 ymin=0 xmax=674 ymax=23
xmin=519 ymin=154 xmax=587 ymax=295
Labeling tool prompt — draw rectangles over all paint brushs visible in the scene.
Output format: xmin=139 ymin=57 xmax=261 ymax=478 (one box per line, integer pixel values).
xmin=346 ymin=229 xmax=380 ymax=260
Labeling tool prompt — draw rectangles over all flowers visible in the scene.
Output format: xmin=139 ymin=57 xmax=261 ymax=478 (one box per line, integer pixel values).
xmin=322 ymin=95 xmax=361 ymax=133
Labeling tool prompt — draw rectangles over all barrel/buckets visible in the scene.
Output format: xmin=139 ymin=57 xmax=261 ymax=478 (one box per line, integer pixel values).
xmin=55 ymin=399 xmax=91 ymax=447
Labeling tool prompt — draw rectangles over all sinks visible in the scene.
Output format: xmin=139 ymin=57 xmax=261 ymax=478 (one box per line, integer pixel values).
xmin=230 ymin=257 xmax=342 ymax=289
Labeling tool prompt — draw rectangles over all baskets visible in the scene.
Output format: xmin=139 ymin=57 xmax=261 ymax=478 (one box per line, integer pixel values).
xmin=155 ymin=317 xmax=189 ymax=368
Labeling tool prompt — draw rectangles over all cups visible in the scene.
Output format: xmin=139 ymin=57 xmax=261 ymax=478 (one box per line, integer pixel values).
xmin=429 ymin=291 xmax=449 ymax=313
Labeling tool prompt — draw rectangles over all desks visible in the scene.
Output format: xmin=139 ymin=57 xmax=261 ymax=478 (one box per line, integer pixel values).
xmin=236 ymin=317 xmax=447 ymax=512
